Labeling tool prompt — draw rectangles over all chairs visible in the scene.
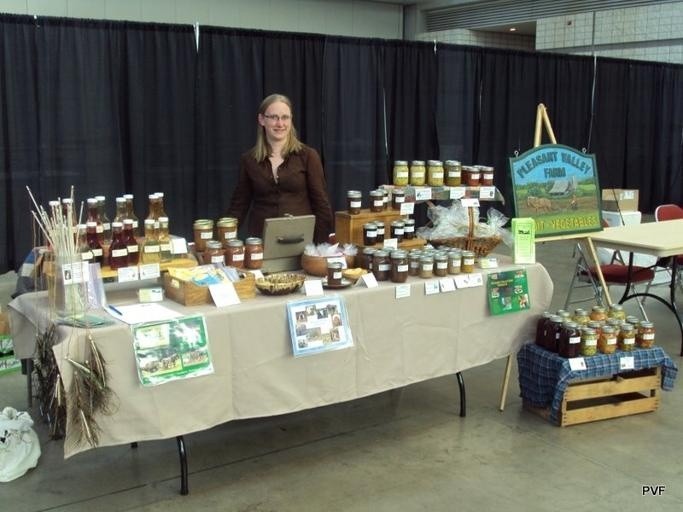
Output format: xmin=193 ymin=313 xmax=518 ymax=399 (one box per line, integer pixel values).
xmin=640 ymin=204 xmax=683 ymax=305
xmin=564 ymin=219 xmax=654 ymax=321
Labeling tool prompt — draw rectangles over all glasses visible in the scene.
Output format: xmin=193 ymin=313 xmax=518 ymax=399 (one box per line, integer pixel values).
xmin=262 ymin=114 xmax=292 ymax=121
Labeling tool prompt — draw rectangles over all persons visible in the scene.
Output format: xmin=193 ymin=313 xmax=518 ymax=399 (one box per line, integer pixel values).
xmin=226 ymin=94 xmax=334 ymax=247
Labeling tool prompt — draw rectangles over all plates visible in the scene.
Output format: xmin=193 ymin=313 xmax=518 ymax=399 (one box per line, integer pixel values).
xmin=318 ymin=279 xmax=352 ymax=288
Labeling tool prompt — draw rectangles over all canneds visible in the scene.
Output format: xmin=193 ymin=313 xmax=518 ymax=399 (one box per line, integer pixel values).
xmin=363 ymin=245 xmax=475 ymax=283
xmin=537 ymin=303 xmax=657 ymax=358
xmin=391 ymin=190 xmax=405 ymax=210
xmin=204 ymin=237 xmax=263 ymax=269
xmin=192 ymin=219 xmax=214 ymax=251
xmin=393 ymin=159 xmax=495 ymax=187
xmin=346 ymin=191 xmax=363 ymax=214
xmin=390 ymin=218 xmax=414 ymax=243
xmin=369 ymin=188 xmax=388 ymax=214
xmin=327 ymin=262 xmax=342 ymax=287
xmin=216 ymin=217 xmax=239 ymax=248
xmin=362 ymin=220 xmax=384 ymax=246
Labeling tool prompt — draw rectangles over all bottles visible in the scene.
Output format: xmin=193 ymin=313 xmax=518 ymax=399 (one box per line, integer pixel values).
xmin=42 ymin=184 xmax=176 ymax=270
xmin=534 ymin=302 xmax=656 ymax=359
xmin=324 ymin=261 xmax=344 ymax=286
xmin=193 ymin=215 xmax=266 ymax=271
xmin=348 ymin=156 xmax=494 ymax=285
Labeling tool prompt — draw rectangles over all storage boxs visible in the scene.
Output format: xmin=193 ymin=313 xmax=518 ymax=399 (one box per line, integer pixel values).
xmin=601 ymin=188 xmax=639 ymax=212
xmin=601 ymin=210 xmax=642 ymax=227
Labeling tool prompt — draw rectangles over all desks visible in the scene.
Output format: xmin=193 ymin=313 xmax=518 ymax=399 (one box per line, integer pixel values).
xmin=7 ymin=251 xmax=554 ymax=495
xmin=590 ymin=219 xmax=683 ymax=357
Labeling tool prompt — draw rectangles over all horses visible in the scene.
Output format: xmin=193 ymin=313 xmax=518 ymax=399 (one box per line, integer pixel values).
xmin=526 ymin=196 xmax=553 ymax=213
